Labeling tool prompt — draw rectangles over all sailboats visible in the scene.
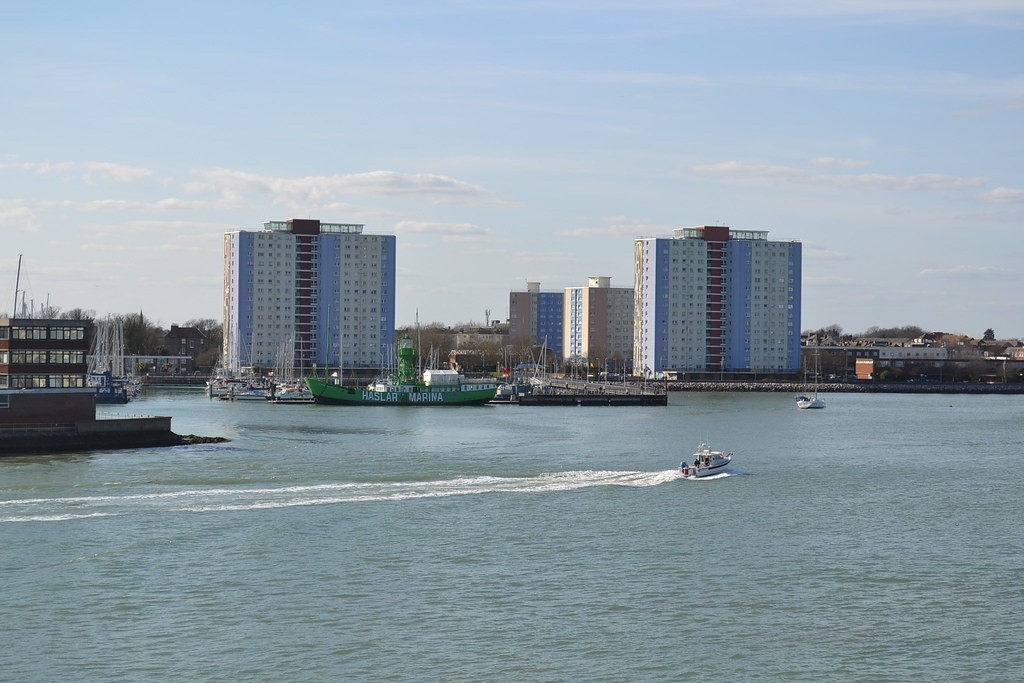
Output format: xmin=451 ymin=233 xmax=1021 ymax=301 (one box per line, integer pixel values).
xmin=81 ymin=312 xmax=145 ymax=403
xmin=203 ymin=315 xmax=669 ymax=407
xmin=792 ymin=339 xmax=827 ymax=409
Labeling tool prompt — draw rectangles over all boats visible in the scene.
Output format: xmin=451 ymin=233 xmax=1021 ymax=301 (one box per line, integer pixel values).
xmin=678 ymin=429 xmax=734 ymax=478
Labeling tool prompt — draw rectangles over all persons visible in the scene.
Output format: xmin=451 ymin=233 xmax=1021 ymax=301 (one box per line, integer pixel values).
xmin=694 ymin=459 xmax=700 ymax=469
